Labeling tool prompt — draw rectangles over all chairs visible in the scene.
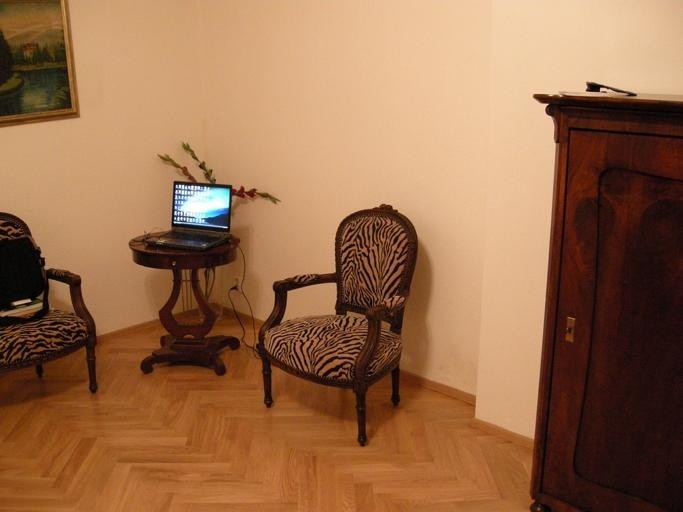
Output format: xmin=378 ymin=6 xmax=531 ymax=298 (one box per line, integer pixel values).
xmin=255 ymin=203 xmax=421 ymax=447
xmin=0 ymin=210 xmax=100 ymax=398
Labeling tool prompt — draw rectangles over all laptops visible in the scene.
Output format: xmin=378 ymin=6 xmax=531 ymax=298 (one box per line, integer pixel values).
xmin=155 ymin=181 xmax=232 ymax=251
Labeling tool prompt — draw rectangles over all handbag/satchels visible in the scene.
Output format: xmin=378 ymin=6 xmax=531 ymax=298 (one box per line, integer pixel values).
xmin=0 ymin=237 xmax=45 ymax=310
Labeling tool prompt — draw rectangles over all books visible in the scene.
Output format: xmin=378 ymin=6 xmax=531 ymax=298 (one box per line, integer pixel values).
xmin=7 ymin=307 xmax=42 ymax=318
xmin=1 ymin=297 xmax=43 ymax=317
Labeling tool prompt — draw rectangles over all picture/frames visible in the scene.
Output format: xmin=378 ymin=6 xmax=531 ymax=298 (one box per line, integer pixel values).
xmin=1 ymin=0 xmax=84 ymax=125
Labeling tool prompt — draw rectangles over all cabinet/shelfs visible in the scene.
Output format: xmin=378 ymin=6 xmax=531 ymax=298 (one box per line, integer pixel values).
xmin=530 ymin=86 xmax=682 ymax=511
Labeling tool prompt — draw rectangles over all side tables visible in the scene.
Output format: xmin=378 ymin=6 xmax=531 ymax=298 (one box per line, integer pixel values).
xmin=128 ymin=226 xmax=244 ymax=377
